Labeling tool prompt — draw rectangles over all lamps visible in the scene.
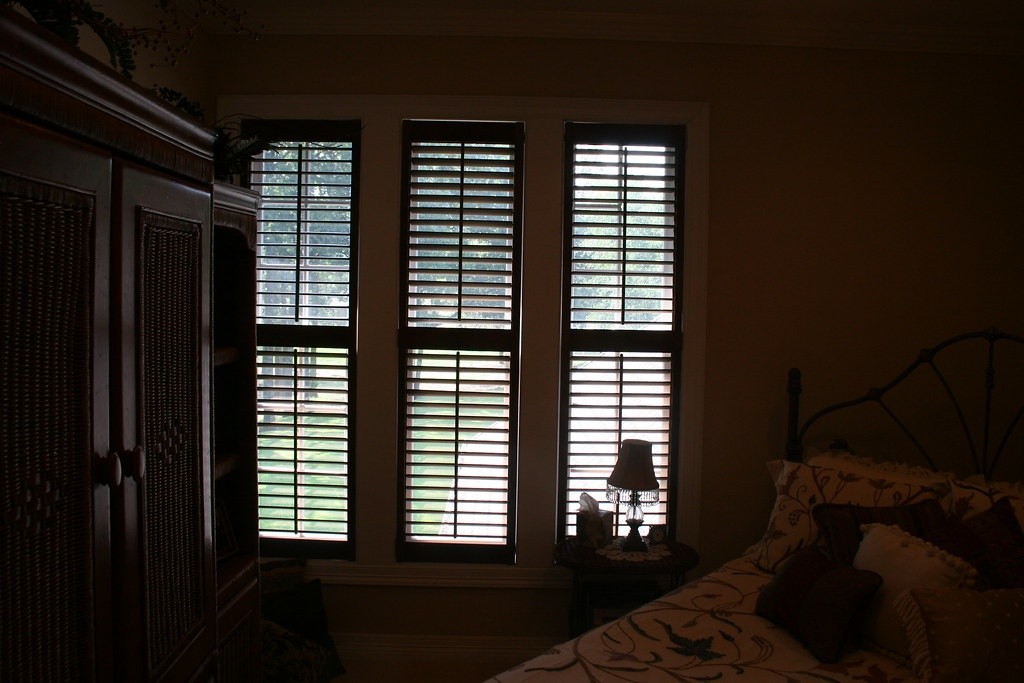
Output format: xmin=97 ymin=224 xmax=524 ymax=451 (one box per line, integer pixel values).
xmin=602 ymin=439 xmax=661 ymax=555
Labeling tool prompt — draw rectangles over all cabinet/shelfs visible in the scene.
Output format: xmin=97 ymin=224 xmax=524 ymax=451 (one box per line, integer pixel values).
xmin=210 ymin=180 xmax=264 ymax=683
xmin=0 ymin=8 xmax=219 ymax=683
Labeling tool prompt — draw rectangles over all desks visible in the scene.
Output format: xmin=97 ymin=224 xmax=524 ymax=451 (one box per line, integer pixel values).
xmin=552 ymin=534 xmax=700 ymax=640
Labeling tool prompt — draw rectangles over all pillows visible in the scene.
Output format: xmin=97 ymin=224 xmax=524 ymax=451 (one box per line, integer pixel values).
xmin=753 ymin=450 xmax=1024 ymax=683
xmin=263 ymin=621 xmax=333 ymax=683
xmin=261 ymin=578 xmax=346 ymax=680
xmin=260 ymin=558 xmax=307 ymax=592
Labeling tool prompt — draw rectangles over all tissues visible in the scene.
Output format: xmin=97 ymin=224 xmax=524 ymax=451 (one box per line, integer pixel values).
xmin=575 ymin=492 xmax=614 ymax=547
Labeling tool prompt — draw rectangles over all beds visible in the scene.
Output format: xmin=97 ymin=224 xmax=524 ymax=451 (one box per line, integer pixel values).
xmin=484 ymin=326 xmax=1024 ymax=683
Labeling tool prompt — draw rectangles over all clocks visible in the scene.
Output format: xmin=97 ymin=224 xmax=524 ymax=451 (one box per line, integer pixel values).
xmin=646 ymin=525 xmax=666 ymax=545
